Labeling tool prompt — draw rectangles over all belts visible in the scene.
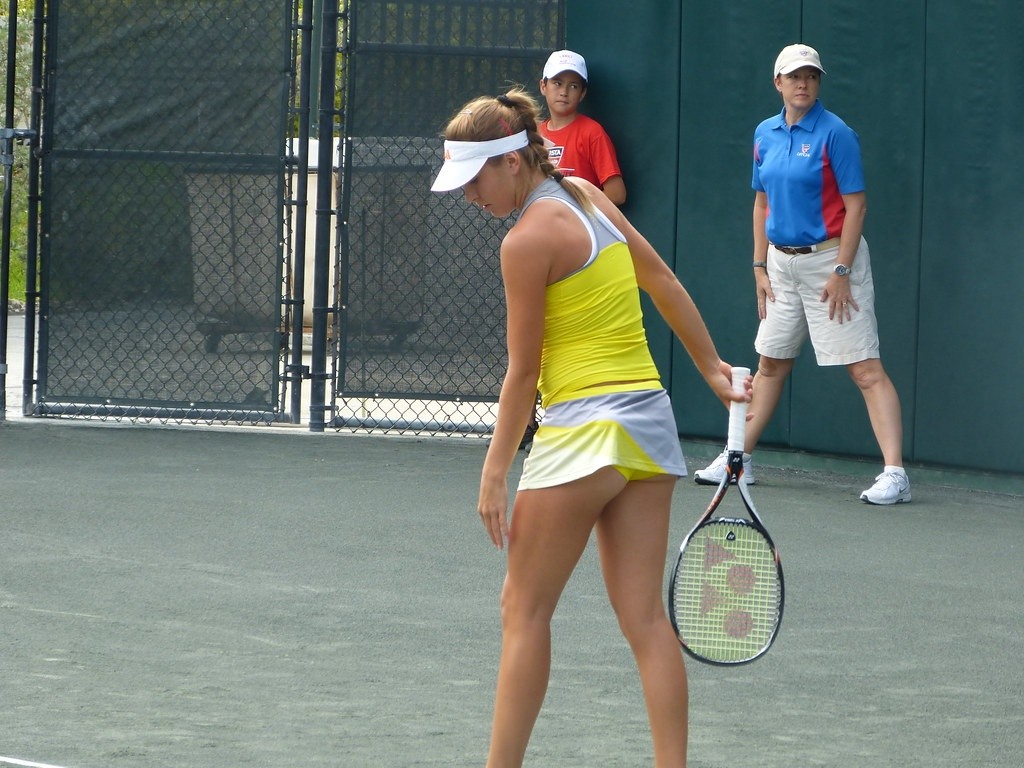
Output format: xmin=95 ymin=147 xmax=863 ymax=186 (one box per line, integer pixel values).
xmin=775 ymin=237 xmax=841 ymax=255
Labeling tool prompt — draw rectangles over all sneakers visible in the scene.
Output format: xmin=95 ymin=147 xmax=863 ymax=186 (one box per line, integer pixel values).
xmin=861 ymin=471 xmax=911 ymax=504
xmin=695 ymin=445 xmax=755 ymax=484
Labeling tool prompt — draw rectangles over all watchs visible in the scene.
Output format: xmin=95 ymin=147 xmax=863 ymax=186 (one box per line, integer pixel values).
xmin=832 ymin=263 xmax=852 ymax=276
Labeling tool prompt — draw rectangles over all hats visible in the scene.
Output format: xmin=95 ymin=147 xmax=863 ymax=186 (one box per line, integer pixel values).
xmin=774 ymin=44 xmax=827 ymax=80
xmin=430 ymin=129 xmax=529 ymax=192
xmin=543 ymin=50 xmax=588 ymax=82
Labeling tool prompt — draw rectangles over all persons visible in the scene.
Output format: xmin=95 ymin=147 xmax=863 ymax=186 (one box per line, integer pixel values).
xmin=485 ymin=50 xmax=628 ymax=454
xmin=693 ymin=42 xmax=912 ymax=505
xmin=430 ymin=89 xmax=755 ymax=768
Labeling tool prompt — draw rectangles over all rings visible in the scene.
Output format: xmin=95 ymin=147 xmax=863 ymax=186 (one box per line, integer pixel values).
xmin=842 ymin=303 xmax=847 ymax=305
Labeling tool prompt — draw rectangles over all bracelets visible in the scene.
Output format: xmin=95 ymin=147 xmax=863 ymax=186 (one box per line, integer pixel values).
xmin=752 ymin=261 xmax=766 ymax=268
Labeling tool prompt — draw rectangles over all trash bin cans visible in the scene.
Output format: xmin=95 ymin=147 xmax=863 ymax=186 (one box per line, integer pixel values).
xmin=181 ymin=129 xmax=447 ymax=357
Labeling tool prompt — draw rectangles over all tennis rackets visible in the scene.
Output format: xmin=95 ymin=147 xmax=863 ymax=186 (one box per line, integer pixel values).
xmin=667 ymin=367 xmax=786 ymax=670
